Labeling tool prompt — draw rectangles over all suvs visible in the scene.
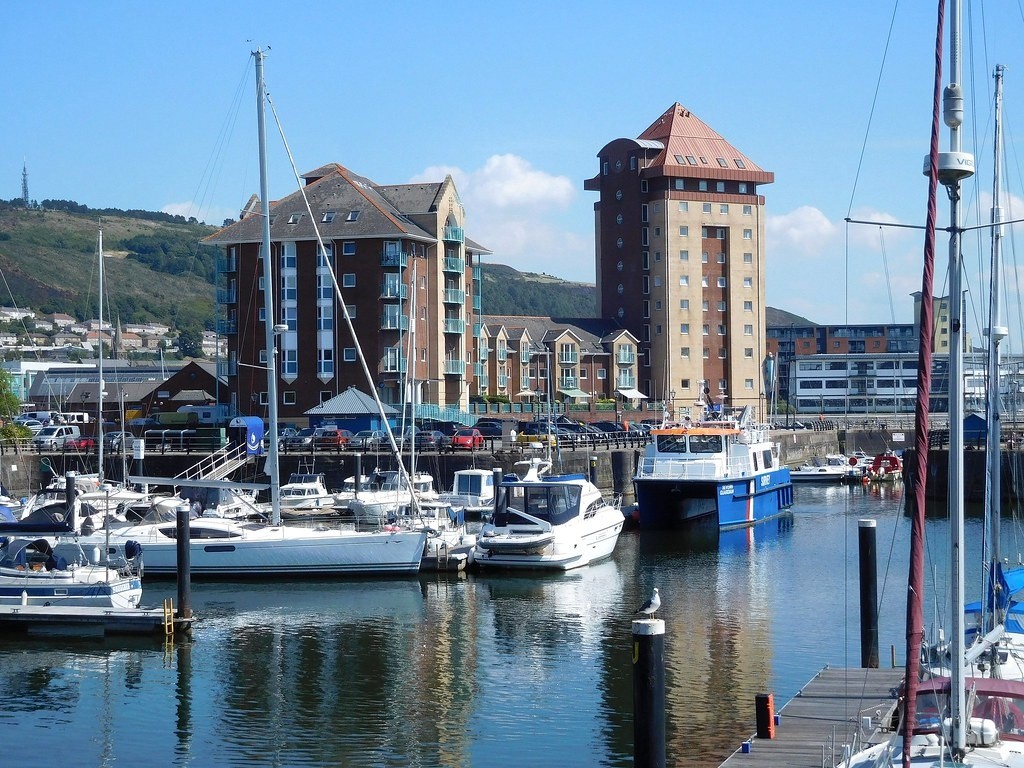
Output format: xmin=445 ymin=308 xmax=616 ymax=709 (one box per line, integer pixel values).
xmin=378 ymin=425 xmax=420 ymax=451
xmin=286 ymin=427 xmax=326 ymax=452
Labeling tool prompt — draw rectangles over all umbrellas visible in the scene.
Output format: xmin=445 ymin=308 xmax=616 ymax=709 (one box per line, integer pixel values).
xmin=515 ymin=389 xmax=537 ymax=403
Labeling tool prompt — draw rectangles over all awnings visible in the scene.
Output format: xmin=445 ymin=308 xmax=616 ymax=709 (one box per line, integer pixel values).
xmin=557 ymin=388 xmax=592 ymax=398
xmin=617 ymin=388 xmax=649 ymax=399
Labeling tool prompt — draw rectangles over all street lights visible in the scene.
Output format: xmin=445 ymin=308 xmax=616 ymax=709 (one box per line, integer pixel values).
xmin=670 ymin=388 xmax=676 ymax=420
xmin=759 ymin=392 xmax=764 ymax=423
xmin=80 ymin=391 xmax=86 ymax=436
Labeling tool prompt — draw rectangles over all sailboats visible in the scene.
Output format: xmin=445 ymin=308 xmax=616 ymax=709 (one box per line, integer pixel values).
xmin=817 ymin=0 xmax=1024 ymax=767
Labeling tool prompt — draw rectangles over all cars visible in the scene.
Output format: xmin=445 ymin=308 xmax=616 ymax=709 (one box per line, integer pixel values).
xmin=66 ymin=435 xmax=94 ymax=454
xmin=129 ymin=418 xmax=154 ymax=425
xmin=263 ymin=428 xmax=297 ymax=451
xmin=96 ymin=431 xmax=134 ymax=452
xmin=414 ymin=431 xmax=451 ymax=451
xmin=321 ymin=429 xmax=354 ymax=451
xmin=16 ymin=419 xmax=43 ymax=435
xmin=348 ymin=430 xmax=384 ymax=452
xmin=629 ymin=418 xmax=662 ymax=438
xmin=452 ymin=429 xmax=484 ymax=452
xmin=470 ymin=422 xmax=503 ymax=439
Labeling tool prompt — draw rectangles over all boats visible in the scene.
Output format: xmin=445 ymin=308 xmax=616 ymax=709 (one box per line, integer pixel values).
xmin=631 ymin=379 xmax=795 ymax=531
xmin=0 ymin=33 xmax=626 ymax=609
xmin=789 ymin=462 xmax=847 ymax=481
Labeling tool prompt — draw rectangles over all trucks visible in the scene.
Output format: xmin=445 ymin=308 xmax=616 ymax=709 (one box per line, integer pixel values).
xmin=148 ymin=411 xmax=198 ymax=425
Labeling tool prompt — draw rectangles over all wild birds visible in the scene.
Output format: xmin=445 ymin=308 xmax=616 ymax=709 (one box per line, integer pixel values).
xmin=634 ymin=587 xmax=662 ymax=620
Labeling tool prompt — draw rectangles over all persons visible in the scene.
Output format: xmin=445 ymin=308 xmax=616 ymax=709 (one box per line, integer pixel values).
xmin=510 ymin=428 xmax=517 ymax=450
xmin=819 ymin=413 xmax=825 ymax=423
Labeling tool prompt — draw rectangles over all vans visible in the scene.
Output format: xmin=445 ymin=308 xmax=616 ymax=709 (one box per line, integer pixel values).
xmin=30 ymin=425 xmax=82 ymax=452
xmin=12 ymin=410 xmax=58 ymax=425
xmin=51 ymin=411 xmax=90 ymax=425
